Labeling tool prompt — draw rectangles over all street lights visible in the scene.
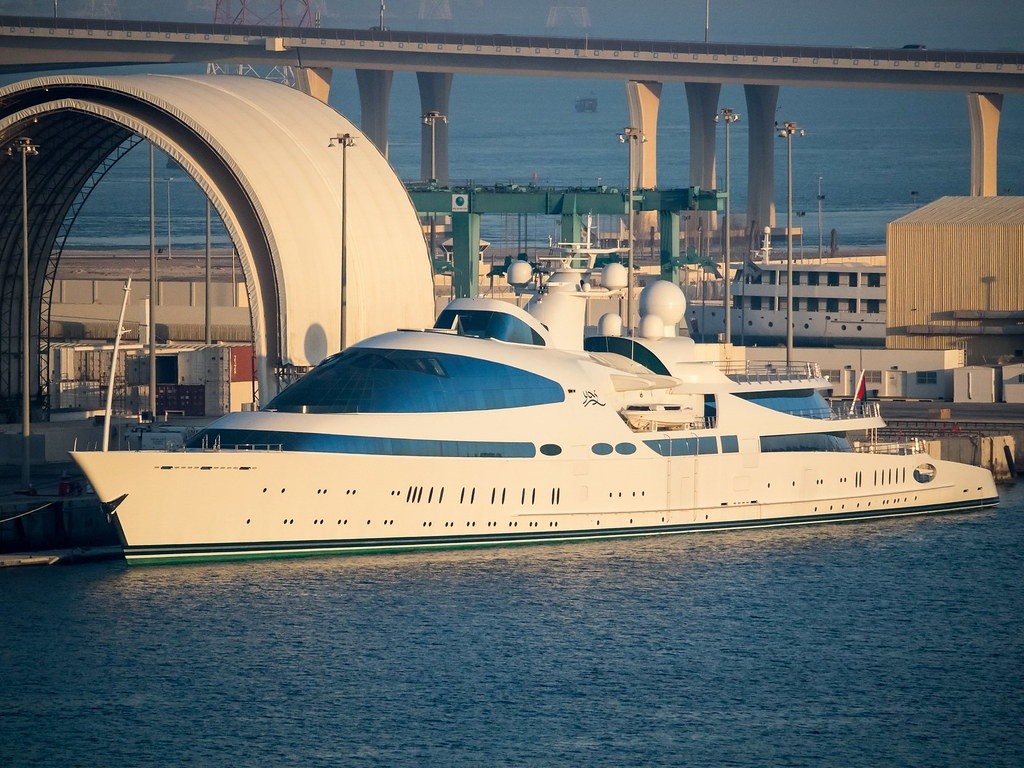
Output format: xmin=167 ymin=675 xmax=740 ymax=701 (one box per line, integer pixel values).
xmin=775 ymin=119 xmax=807 ymax=366
xmin=817 ymin=176 xmax=825 ymax=259
xmin=7 ymin=136 xmax=41 ymax=487
xmin=328 ymin=133 xmax=360 ymax=352
xmin=422 ymin=109 xmax=449 ymax=182
xmin=714 ymin=107 xmax=741 ymax=344
xmin=618 ymin=126 xmax=648 ymax=338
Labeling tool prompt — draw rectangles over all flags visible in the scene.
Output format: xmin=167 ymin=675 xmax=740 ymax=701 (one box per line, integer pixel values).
xmin=857 ymin=374 xmax=867 ymax=414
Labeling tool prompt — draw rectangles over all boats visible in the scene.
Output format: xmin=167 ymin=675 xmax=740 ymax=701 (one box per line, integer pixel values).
xmin=682 ymin=260 xmax=888 ymax=349
xmin=70 ymin=242 xmax=1001 ymax=565
